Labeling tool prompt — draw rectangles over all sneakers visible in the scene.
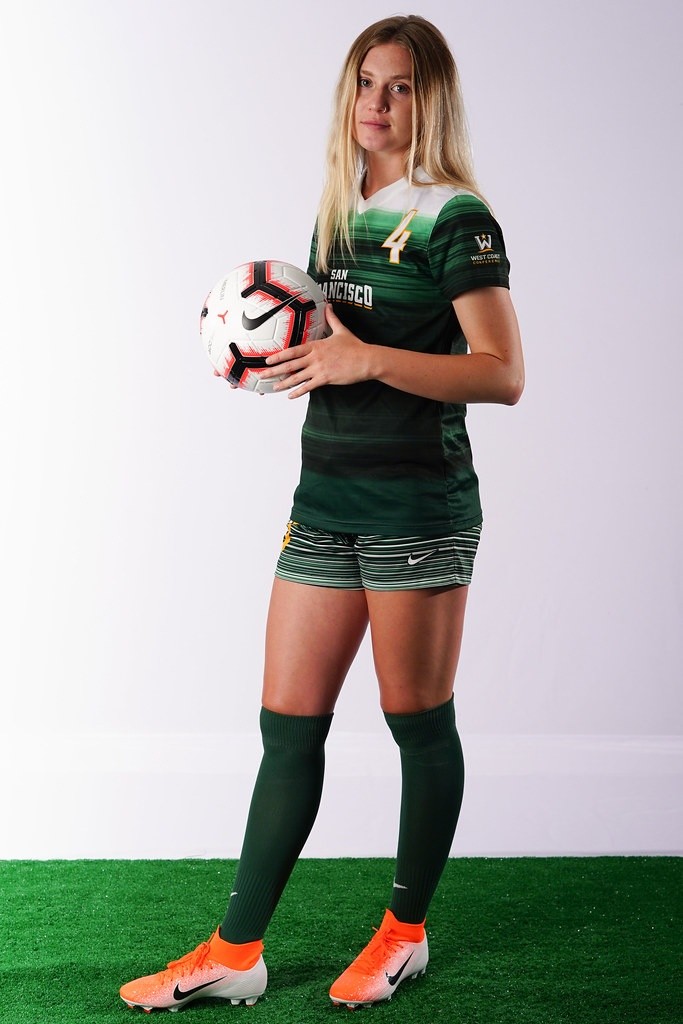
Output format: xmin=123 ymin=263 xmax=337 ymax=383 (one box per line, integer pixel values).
xmin=329 ymin=908 xmax=429 ymax=1007
xmin=119 ymin=924 xmax=268 ymax=1011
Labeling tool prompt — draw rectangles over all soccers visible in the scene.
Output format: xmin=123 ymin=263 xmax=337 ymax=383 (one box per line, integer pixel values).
xmin=200 ymin=259 xmax=331 ymax=394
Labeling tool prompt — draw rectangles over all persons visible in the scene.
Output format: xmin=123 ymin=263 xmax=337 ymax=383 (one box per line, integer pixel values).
xmin=117 ymin=12 xmax=525 ymax=1010
